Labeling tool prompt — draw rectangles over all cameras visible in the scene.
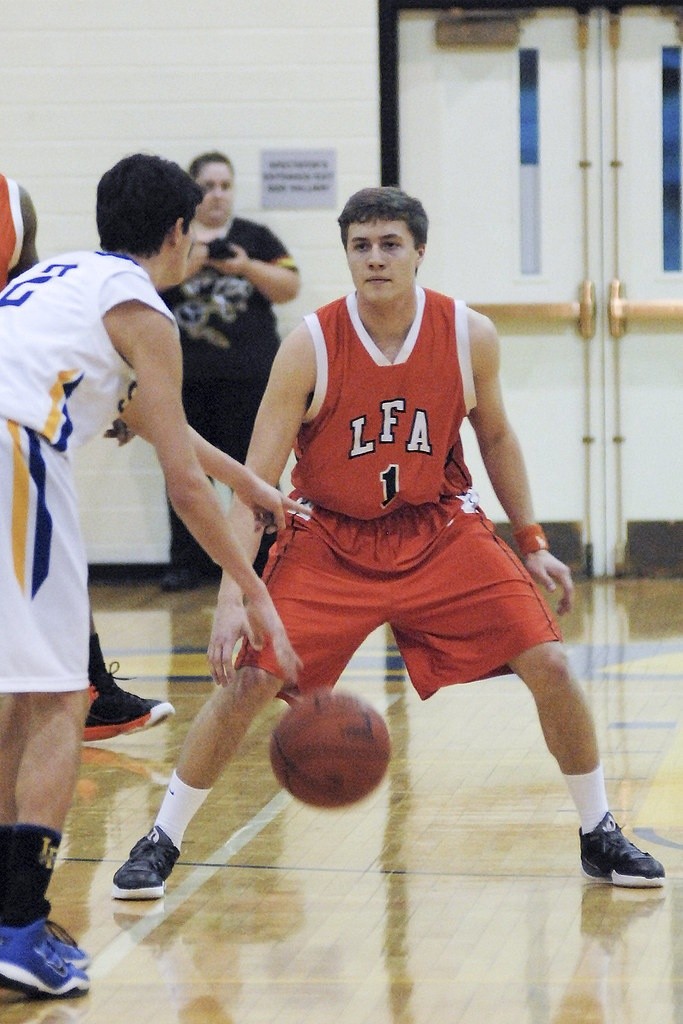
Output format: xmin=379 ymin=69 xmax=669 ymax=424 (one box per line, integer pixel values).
xmin=208 ymin=239 xmax=235 ymax=258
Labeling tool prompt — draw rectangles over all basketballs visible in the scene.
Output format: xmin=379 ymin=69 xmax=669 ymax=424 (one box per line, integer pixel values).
xmin=269 ymin=690 xmax=393 ymax=810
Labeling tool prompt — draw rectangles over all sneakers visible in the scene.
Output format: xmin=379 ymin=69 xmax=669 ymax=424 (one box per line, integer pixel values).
xmin=0 ymin=904 xmax=91 ymax=999
xmin=79 ymin=663 xmax=171 ymax=743
xmin=579 ymin=814 xmax=665 ymax=888
xmin=112 ymin=823 xmax=184 ymax=898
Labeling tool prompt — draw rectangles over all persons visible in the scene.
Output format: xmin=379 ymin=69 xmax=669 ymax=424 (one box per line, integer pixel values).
xmin=0 ymin=155 xmax=308 ymax=999
xmin=111 ymin=188 xmax=665 ymax=901
xmin=157 ymin=153 xmax=300 ymax=592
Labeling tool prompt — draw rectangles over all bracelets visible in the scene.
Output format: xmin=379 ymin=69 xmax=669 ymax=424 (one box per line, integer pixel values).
xmin=515 ymin=524 xmax=549 ymax=555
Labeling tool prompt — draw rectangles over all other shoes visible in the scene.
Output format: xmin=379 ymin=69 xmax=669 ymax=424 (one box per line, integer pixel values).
xmin=158 ymin=563 xmax=214 ymax=590
